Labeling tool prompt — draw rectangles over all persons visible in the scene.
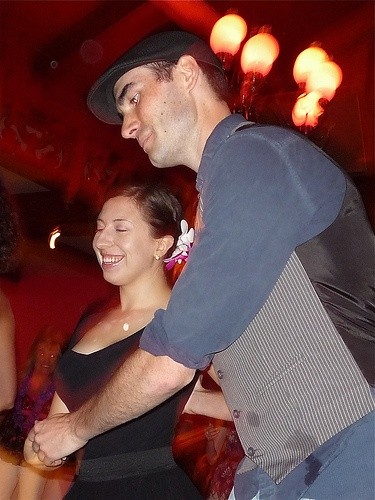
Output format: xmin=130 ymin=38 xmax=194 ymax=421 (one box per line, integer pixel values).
xmin=0 ymin=28 xmax=375 ymax=500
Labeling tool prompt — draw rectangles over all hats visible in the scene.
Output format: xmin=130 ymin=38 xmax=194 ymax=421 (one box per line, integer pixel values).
xmin=87 ymin=30 xmax=228 ymax=125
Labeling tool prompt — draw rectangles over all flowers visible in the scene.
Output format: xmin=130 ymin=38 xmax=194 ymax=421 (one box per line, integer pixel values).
xmin=163 ymin=220 xmax=199 ymax=275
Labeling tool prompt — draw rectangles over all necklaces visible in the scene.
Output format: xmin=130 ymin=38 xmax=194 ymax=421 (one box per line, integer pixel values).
xmin=114 ymin=303 xmax=164 ymax=332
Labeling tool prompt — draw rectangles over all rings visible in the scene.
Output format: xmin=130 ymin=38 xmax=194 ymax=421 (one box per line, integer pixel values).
xmin=206 ymin=431 xmax=209 ymax=434
